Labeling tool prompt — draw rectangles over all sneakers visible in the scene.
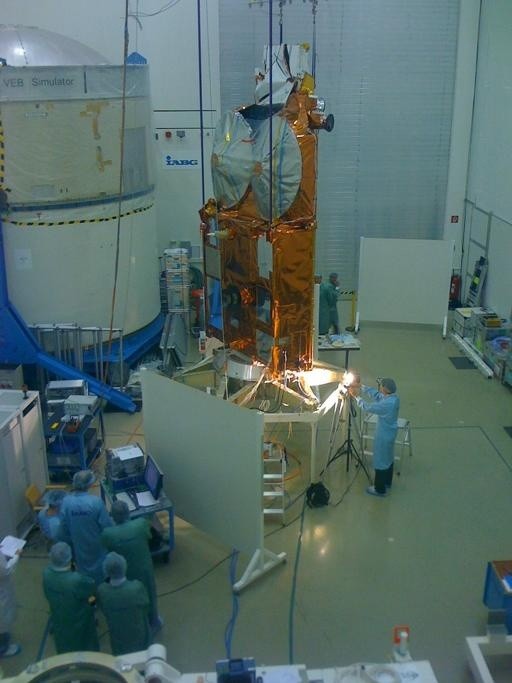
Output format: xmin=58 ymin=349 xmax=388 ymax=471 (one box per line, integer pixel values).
xmin=3 ymin=643 xmax=18 ymax=657
xmin=367 ymin=485 xmax=385 ymax=496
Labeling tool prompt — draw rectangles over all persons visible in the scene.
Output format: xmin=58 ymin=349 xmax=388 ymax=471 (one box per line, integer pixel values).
xmin=101 ymin=498 xmax=166 ymax=639
xmin=58 ymin=469 xmax=114 ymax=583
xmin=0 ymin=544 xmax=22 ymax=659
xmin=36 ymin=489 xmax=78 ymax=573
xmin=95 ymin=550 xmax=151 ymax=656
xmin=315 ymin=270 xmax=334 ymax=335
xmin=347 ymin=376 xmax=401 ymax=497
xmin=325 ymin=271 xmax=343 ymax=334
xmin=40 ymin=540 xmax=101 ymax=682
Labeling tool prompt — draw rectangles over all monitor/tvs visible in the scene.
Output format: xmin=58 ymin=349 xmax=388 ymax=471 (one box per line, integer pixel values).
xmin=142 ymin=453 xmax=164 ymax=500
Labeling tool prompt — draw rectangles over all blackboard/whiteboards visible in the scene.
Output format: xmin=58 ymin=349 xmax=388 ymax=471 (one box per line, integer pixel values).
xmin=358 ymin=235 xmax=454 ymax=324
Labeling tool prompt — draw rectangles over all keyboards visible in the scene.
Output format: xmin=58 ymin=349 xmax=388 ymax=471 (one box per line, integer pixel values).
xmin=115 ymin=492 xmax=137 ymax=512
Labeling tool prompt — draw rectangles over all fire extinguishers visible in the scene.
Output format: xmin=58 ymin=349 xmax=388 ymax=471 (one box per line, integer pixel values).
xmin=451 ymin=273 xmax=461 ymax=300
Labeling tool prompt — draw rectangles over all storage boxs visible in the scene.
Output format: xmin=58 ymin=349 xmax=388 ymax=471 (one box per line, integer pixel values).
xmin=454 ymin=307 xmax=512 ymax=388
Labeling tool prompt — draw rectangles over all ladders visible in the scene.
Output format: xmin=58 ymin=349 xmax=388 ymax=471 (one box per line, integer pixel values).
xmin=463 ymin=260 xmax=488 ymax=306
xmin=262 ymin=438 xmax=285 ymax=527
